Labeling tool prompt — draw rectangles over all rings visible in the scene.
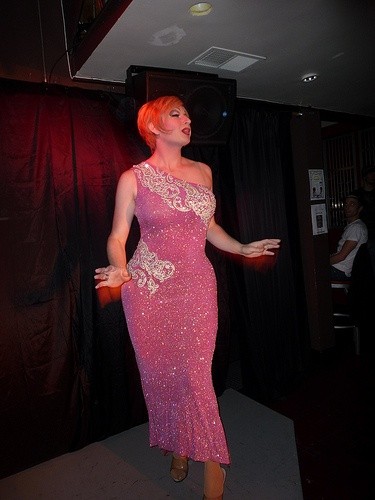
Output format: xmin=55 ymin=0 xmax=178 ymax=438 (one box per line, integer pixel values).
xmin=104 ymin=274 xmax=109 ymax=281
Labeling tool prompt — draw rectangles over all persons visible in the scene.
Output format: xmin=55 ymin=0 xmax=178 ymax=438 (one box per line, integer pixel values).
xmin=94 ymin=95 xmax=281 ymax=500
xmin=330 ymin=195 xmax=368 ymax=280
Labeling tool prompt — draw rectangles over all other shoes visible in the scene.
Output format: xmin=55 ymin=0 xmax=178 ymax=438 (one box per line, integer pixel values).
xmin=170 ymin=452 xmax=188 ymax=482
xmin=201 ymin=467 xmax=227 ymax=500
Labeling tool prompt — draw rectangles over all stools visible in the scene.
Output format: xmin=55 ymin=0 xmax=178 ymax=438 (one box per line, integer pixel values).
xmin=330 ymin=280 xmax=361 ymax=355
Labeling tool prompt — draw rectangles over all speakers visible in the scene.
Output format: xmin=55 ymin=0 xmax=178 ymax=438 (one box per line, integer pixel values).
xmin=125 ymin=65 xmax=237 ymax=145
